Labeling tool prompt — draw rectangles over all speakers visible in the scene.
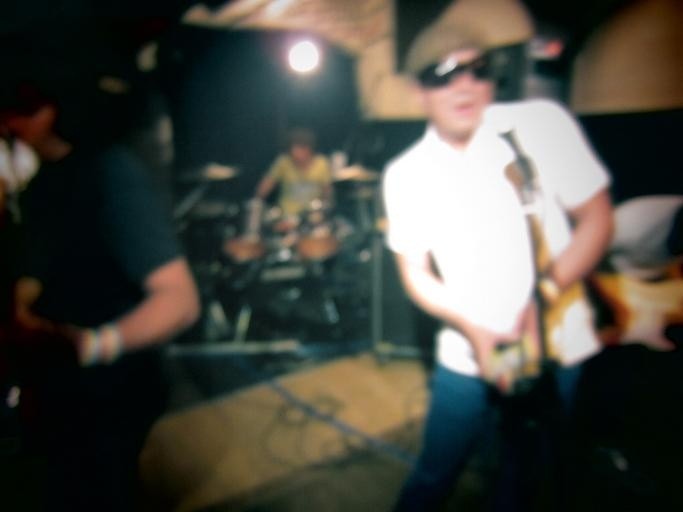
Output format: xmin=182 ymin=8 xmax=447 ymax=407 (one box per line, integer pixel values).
xmin=371 ymin=235 xmax=441 ymax=356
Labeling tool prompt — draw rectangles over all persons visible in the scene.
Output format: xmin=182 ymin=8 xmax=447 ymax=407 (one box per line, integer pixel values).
xmin=254 ymin=127 xmax=333 ymax=216
xmin=0 ymin=51 xmax=200 ymax=512
xmin=380 ymin=22 xmax=616 ymax=512
xmin=604 ymin=193 xmax=683 ymax=270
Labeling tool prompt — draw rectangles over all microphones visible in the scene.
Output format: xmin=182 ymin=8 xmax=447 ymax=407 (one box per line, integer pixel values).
xmin=497 ymin=115 xmax=535 ymax=192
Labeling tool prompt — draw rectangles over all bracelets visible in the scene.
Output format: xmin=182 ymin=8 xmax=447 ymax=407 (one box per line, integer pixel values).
xmin=533 ymin=273 xmax=560 ymax=308
xmin=79 ymin=322 xmax=120 ymax=368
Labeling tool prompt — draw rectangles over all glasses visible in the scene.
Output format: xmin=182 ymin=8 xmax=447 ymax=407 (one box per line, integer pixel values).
xmin=421 ymin=51 xmax=505 ymax=84
